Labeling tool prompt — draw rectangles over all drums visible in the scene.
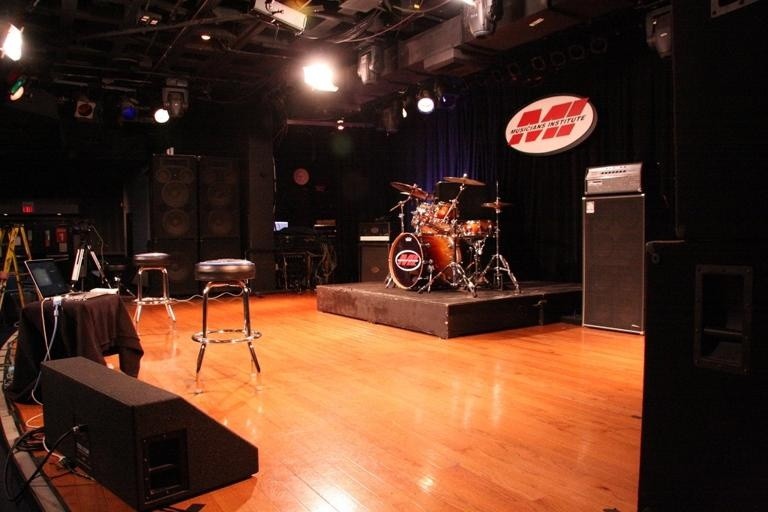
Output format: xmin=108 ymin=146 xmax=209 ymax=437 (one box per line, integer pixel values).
xmin=461 ymin=220 xmax=495 ymax=237
xmin=410 ymin=202 xmax=463 ymax=235
xmin=388 ymin=232 xmax=461 ymax=291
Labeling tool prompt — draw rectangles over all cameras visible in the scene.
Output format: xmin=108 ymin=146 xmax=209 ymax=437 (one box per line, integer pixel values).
xmin=72 ymin=222 xmax=89 ymax=236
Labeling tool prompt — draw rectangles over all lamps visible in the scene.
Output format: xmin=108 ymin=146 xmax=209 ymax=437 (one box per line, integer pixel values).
xmin=467 ymin=0 xmax=496 ymax=39
xmin=416 ymin=86 xmax=436 ymax=112
xmin=356 ymin=44 xmax=381 ymax=85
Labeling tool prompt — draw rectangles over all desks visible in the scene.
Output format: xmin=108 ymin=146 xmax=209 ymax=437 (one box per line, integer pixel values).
xmin=6 ymin=290 xmax=144 ymax=406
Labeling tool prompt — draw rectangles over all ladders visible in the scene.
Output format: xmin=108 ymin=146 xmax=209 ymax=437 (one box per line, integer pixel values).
xmin=0 ymin=223 xmax=44 ymax=312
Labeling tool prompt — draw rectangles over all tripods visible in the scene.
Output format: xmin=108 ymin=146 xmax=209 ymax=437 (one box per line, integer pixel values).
xmin=383 ymin=184 xmax=520 ymax=298
xmin=71 ymin=237 xmax=112 ymax=291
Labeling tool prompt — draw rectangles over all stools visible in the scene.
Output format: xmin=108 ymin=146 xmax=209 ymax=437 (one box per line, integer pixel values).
xmin=192 ymin=259 xmax=260 ymax=394
xmin=133 ymin=252 xmax=176 ymax=322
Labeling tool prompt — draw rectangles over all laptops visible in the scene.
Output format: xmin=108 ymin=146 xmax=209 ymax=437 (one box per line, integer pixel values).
xmin=24 ymin=258 xmax=107 ymax=299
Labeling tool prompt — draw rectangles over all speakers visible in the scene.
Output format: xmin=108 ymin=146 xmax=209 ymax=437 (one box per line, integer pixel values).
xmin=583 ymin=194 xmax=673 ymax=339
xmin=40 ymin=358 xmax=259 ymax=510
xmin=151 ymin=156 xmax=199 ymax=239
xmin=199 ymin=239 xmax=244 ymax=297
xmin=637 ymin=240 xmax=767 ymax=512
xmin=671 ymin=0 xmax=768 ymax=239
xmin=358 ymin=243 xmax=390 ymax=282
xmin=198 ymin=156 xmax=241 ymax=239
xmin=147 ymin=239 xmax=199 ymax=295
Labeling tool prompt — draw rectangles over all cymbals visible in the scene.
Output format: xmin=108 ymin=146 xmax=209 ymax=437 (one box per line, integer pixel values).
xmin=444 ymin=177 xmax=485 ymax=186
xmin=482 ymin=203 xmax=512 ymax=207
xmin=391 ymin=182 xmax=438 ymax=201
